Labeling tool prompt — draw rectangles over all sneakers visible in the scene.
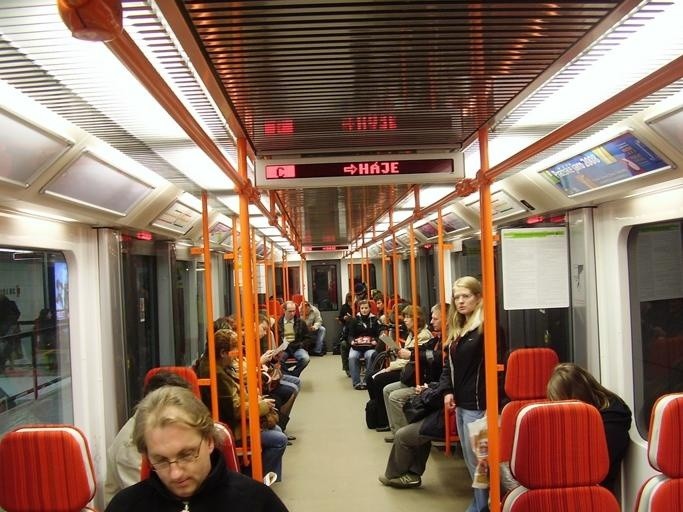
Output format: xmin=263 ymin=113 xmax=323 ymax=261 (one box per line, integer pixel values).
xmin=353 ymin=382 xmax=361 ymax=390
xmin=361 ymin=382 xmax=367 ymax=390
xmin=379 ymin=473 xmax=421 ymax=488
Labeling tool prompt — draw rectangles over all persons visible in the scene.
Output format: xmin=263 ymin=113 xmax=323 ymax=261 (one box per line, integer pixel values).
xmin=0 ymin=293 xmax=54 ymax=410
xmin=105 ymin=372 xmax=288 ymax=512
xmin=335 ymin=277 xmax=485 ymax=490
xmin=549 ymin=363 xmax=630 ymax=484
xmin=200 ymin=300 xmax=326 ymax=488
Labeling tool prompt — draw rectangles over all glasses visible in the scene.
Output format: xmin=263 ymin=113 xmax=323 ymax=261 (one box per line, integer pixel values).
xmin=147 ymin=434 xmax=205 ymax=472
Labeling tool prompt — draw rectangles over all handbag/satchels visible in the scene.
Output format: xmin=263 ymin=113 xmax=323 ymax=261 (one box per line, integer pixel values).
xmin=363 ymin=352 xmax=388 ymax=383
xmin=366 ymin=399 xmax=388 ymax=429
xmin=467 ymin=409 xmax=489 ymax=489
xmin=261 ymin=408 xmax=279 ymax=430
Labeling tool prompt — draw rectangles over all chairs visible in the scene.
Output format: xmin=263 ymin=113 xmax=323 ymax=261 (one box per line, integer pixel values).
xmin=287 ymin=301 xmax=300 ymax=319
xmin=144 ymin=366 xmax=201 ymax=403
xmin=387 ymin=298 xmax=403 ymax=310
xmin=501 ymin=399 xmax=620 ymax=512
xmin=498 ymin=349 xmax=558 ymax=490
xmin=230 ymin=447 xmax=264 ymax=457
xmin=356 ymin=300 xmax=377 ymax=317
xmin=634 ymin=392 xmax=683 ymax=512
xmin=2 ymin=425 xmax=99 ymax=512
xmin=268 ymin=301 xmax=285 ymax=320
xmin=140 ymin=420 xmax=238 ymax=484
xmin=292 ymin=294 xmax=305 ymax=304
xmin=253 ymin=303 xmax=268 ymax=313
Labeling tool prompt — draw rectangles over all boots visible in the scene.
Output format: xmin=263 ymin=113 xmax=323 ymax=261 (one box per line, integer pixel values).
xmin=279 ymin=415 xmax=296 ymax=445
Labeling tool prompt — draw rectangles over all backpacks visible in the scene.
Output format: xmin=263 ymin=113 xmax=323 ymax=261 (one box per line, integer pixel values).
xmin=402 ymin=382 xmax=444 ymax=424
